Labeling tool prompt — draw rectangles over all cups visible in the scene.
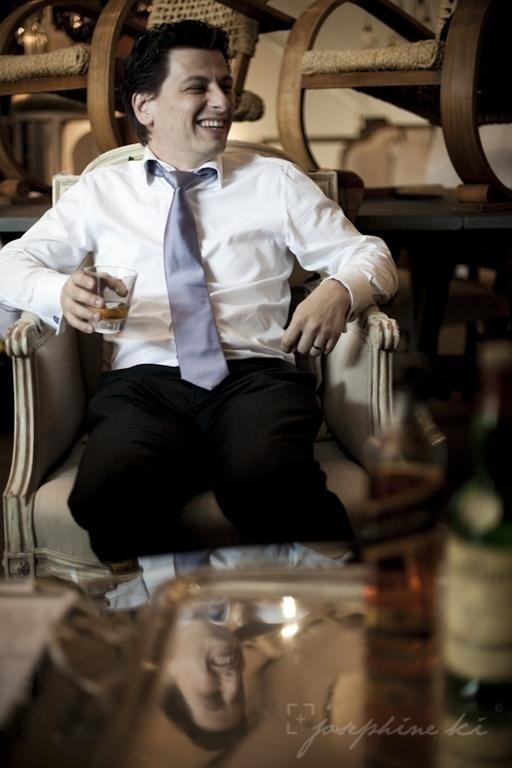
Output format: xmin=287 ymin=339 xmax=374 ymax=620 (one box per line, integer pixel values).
xmin=84 ymin=267 xmax=138 ymax=335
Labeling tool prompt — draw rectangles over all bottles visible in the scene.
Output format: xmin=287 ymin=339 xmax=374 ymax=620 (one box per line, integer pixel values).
xmin=436 ymin=382 xmax=512 ymax=714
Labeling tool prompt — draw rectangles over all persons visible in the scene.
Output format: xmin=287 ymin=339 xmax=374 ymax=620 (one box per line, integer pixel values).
xmin=0 ymin=17 xmax=401 ymax=563
xmin=105 ymin=542 xmax=366 ymax=752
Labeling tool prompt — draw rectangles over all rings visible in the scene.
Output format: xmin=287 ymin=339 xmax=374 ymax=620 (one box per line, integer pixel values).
xmin=313 ymin=344 xmax=324 ymax=351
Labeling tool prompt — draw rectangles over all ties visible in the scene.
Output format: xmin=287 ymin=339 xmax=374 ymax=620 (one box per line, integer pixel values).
xmin=149 ymin=161 xmax=230 ymax=393
